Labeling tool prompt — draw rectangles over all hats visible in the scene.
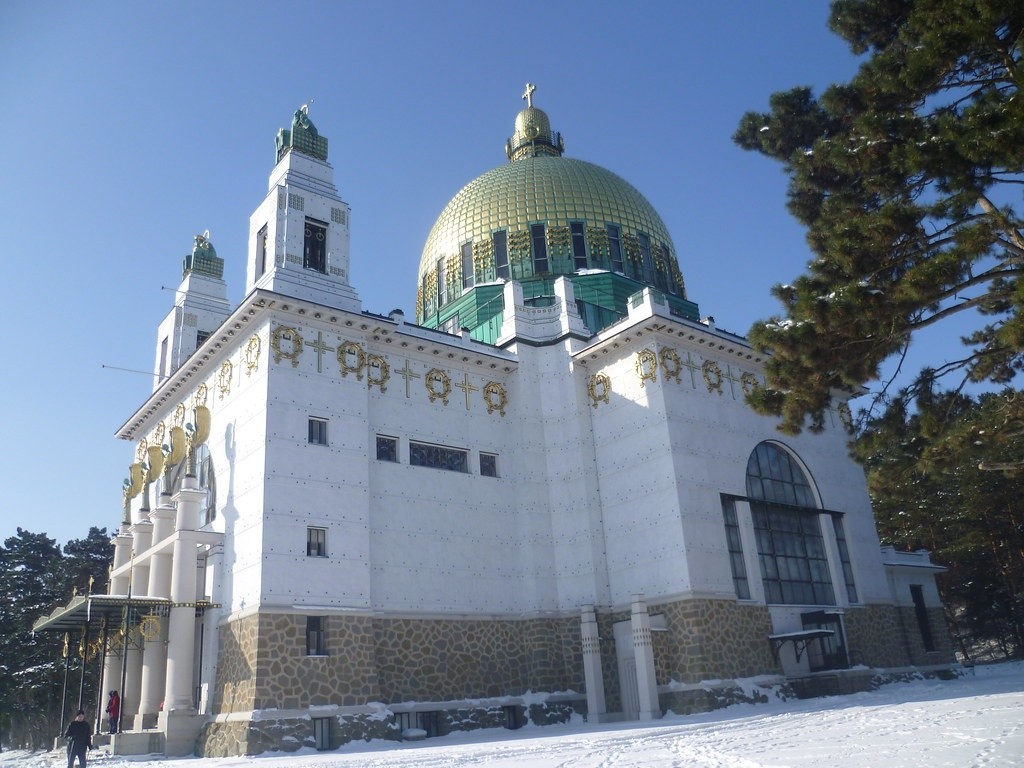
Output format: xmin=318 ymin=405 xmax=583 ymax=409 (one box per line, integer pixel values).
xmin=76 ymin=710 xmax=84 ymax=716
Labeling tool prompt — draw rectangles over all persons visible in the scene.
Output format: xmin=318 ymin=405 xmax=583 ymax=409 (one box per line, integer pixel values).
xmin=122 ymin=479 xmax=131 ymax=523
xmin=161 ymin=444 xmax=171 ymax=491
xmin=185 ymin=423 xmax=197 ymax=475
xmin=64 ymin=710 xmax=92 ymax=768
xmin=141 ymin=462 xmax=150 ymax=508
xmin=106 ymin=690 xmax=120 ymax=734
